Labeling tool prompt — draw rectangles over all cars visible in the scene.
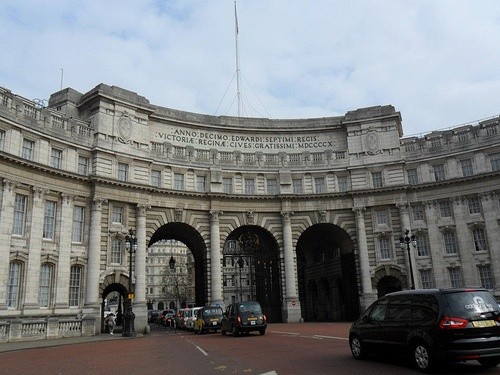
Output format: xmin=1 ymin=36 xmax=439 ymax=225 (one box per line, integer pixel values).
xmin=145 ymin=305 xmax=223 ymax=334
xmin=220 ymin=301 xmax=267 ymax=335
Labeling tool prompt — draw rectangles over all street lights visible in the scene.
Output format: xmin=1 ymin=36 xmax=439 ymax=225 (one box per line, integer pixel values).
xmin=399 ymin=228 xmax=419 ymax=289
xmin=121 ymin=227 xmax=137 ymax=336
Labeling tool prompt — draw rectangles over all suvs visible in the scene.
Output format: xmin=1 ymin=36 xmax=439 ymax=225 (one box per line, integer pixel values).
xmin=347 ymin=286 xmax=500 ymax=371
